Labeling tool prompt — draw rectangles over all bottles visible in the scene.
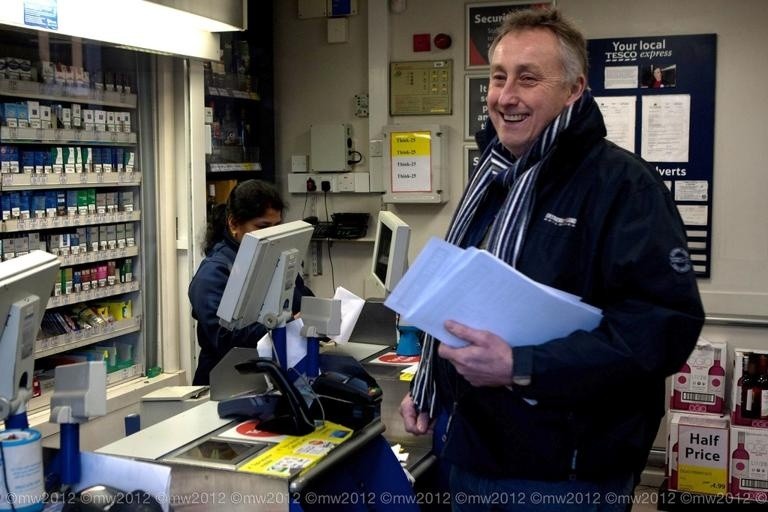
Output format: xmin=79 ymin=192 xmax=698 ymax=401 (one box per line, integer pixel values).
xmin=672 ymin=362 xmax=692 ymax=409
xmin=741 ymin=356 xmax=755 ymax=416
xmin=705 ymin=347 xmax=727 ymax=413
xmin=730 ymin=430 xmax=750 ymax=494
xmin=754 ymin=357 xmax=768 ymax=419
xmin=206 ymin=100 xmax=252 ymax=162
xmin=735 ymin=377 xmax=753 ymax=426
xmin=671 ymin=441 xmax=680 ymax=488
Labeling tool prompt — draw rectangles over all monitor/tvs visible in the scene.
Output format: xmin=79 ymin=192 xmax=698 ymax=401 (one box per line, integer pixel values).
xmin=0 ymin=249 xmax=62 ymax=430
xmin=209 ymin=220 xmax=315 ymax=400
xmin=348 ymin=211 xmax=411 ymax=347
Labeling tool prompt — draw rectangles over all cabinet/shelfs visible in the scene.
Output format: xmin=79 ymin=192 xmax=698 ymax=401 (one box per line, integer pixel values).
xmin=0 ymin=79 xmax=147 ymax=429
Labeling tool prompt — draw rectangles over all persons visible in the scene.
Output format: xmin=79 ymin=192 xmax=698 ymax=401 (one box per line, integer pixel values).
xmin=187 ymin=177 xmax=316 ymax=386
xmin=401 ymin=5 xmax=706 ymax=511
